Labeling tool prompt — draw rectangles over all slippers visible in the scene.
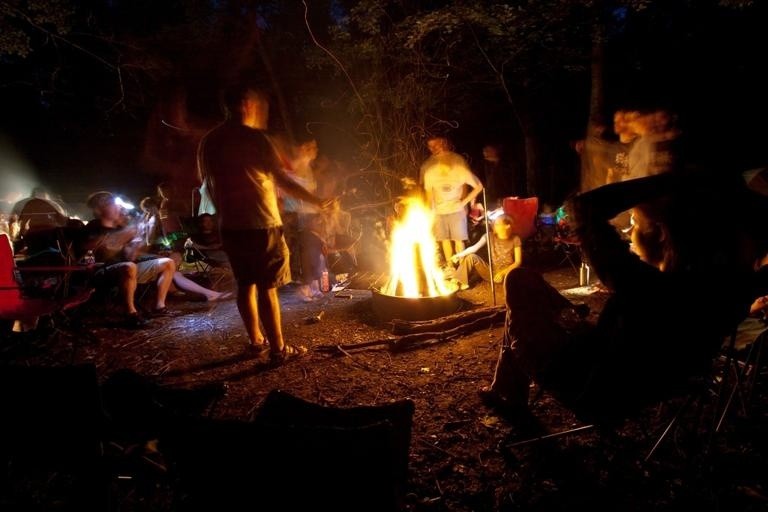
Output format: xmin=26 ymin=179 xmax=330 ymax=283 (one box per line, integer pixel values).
xmin=207 ymin=289 xmax=235 ymax=302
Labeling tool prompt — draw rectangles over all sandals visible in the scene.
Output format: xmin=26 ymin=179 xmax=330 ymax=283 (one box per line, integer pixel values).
xmin=266 ymin=343 xmax=311 ymax=366
xmin=247 ymin=337 xmax=270 ymax=356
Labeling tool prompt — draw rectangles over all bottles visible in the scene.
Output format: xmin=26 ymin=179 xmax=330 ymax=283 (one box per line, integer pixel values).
xmin=183 ymin=238 xmax=194 ymax=263
xmin=579 ymin=261 xmax=590 ymax=286
xmin=319 ymin=255 xmax=330 ymax=291
xmin=83 ymin=250 xmax=95 ymax=265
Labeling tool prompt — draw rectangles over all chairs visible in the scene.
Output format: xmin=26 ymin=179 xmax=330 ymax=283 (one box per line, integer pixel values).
xmin=0 ymin=205 xmax=414 ymax=509
xmin=498 ymin=194 xmax=766 ymax=512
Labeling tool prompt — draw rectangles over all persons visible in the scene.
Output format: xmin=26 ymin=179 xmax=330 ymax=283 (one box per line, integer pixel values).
xmin=473 ymin=171 xmax=765 ymax=418
xmin=197 ymin=82 xmax=335 ymax=366
xmin=452 ymin=214 xmax=523 ymax=296
xmin=420 ymin=133 xmax=484 ymax=290
xmin=277 ymin=131 xmax=332 ymax=296
xmin=6 ymin=186 xmax=222 ymax=323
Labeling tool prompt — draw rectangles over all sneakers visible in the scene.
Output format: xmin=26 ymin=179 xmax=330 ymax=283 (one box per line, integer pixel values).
xmin=126 ymin=310 xmax=156 ymax=327
xmin=153 ymin=306 xmax=184 ymax=315
xmin=478 ymin=384 xmax=530 ymax=412
